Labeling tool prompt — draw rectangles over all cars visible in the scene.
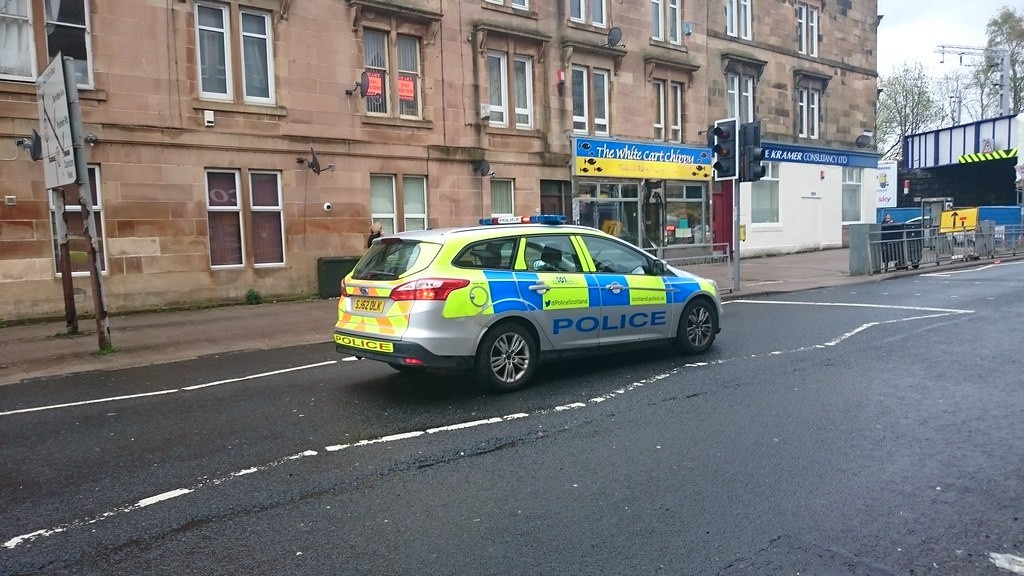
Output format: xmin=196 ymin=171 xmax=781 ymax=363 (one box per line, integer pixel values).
xmin=333 ymin=214 xmax=723 ymax=395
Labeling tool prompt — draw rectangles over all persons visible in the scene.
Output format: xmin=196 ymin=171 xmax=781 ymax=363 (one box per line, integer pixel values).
xmin=368 ymin=222 xmax=385 ymax=249
xmin=882 ymin=214 xmax=896 ymax=224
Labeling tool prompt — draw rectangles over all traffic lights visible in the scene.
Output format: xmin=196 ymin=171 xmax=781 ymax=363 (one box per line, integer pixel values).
xmin=739 ymin=122 xmax=768 ymax=182
xmin=712 ymin=118 xmax=739 ymax=182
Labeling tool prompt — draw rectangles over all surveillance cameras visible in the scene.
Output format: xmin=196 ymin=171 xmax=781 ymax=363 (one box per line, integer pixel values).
xmin=323 ymin=203 xmax=332 ymax=210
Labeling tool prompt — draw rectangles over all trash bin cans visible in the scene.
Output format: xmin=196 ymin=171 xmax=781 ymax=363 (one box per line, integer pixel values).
xmin=881 ymin=222 xmax=922 ymax=270
